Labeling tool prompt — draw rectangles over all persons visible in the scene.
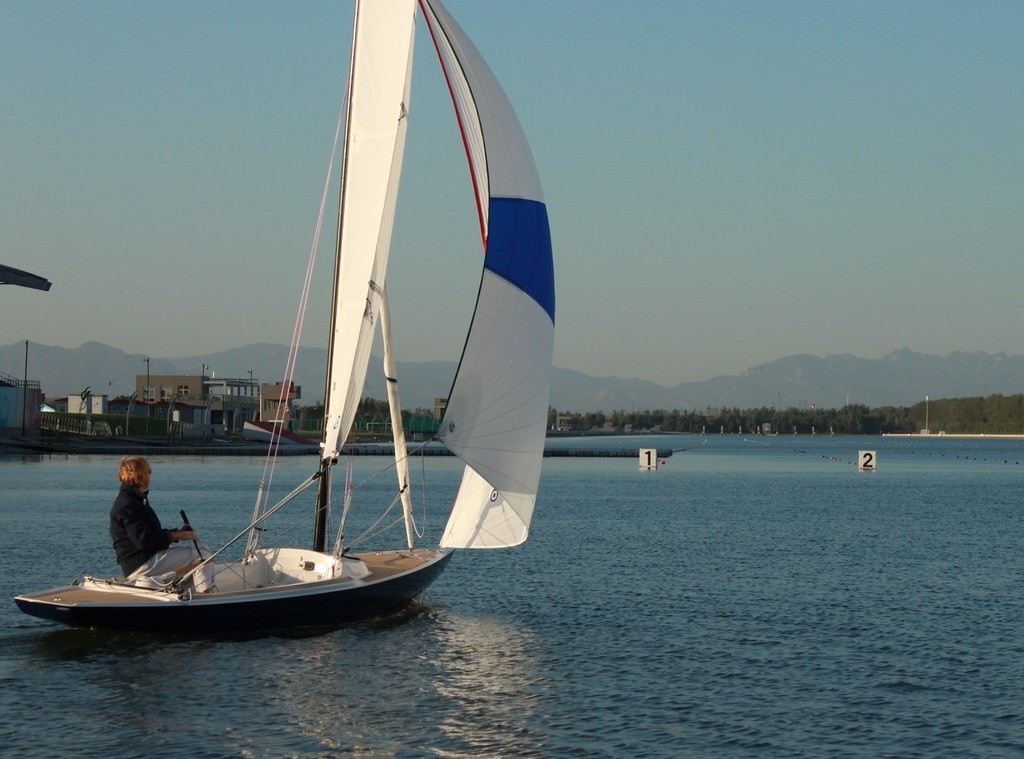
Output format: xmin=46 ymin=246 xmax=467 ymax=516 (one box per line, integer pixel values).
xmin=110 ymin=457 xmax=215 ymax=594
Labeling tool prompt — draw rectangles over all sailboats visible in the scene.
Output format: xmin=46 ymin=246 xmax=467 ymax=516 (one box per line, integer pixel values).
xmin=13 ymin=0 xmax=556 ymax=633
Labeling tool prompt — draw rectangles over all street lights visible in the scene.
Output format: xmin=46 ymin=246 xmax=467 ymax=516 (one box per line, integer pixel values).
xmin=201 ymin=362 xmax=209 ymax=432
xmin=247 ymin=369 xmax=253 ymax=422
xmin=142 ymin=357 xmax=150 ymax=437
